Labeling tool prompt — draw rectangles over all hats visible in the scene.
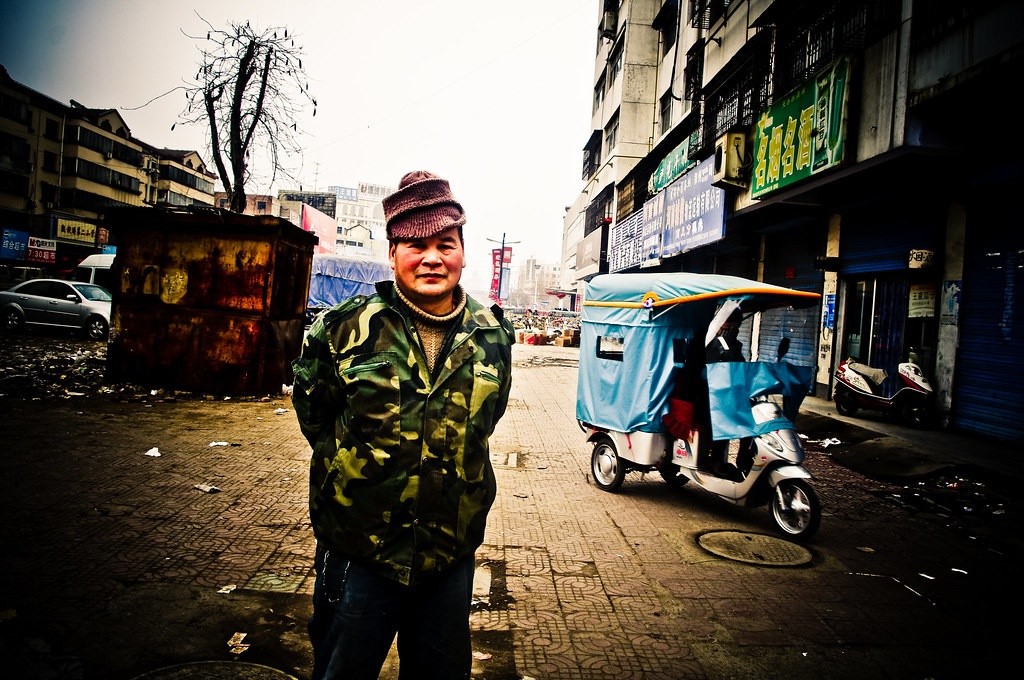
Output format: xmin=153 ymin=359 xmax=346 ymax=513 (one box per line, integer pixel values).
xmin=382 ymin=171 xmax=464 ymax=240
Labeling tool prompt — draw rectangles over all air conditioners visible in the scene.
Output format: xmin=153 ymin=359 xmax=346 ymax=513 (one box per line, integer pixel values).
xmin=603 ymin=11 xmax=614 ymax=32
xmin=714 ymin=132 xmax=747 ymax=181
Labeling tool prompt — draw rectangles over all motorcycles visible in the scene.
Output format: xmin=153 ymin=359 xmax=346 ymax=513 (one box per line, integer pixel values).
xmin=576 ymin=271 xmax=822 ymax=540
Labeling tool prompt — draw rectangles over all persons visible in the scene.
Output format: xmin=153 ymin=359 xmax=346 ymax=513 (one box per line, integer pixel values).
xmin=291 ymin=169 xmax=516 ymax=680
xmin=678 ymin=306 xmax=758 ymax=478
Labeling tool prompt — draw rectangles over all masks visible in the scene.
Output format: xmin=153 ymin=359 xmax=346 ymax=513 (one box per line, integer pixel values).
xmin=716 ymin=329 xmax=740 ymax=340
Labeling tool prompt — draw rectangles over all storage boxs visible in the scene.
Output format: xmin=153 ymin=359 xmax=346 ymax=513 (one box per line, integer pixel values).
xmin=515 ymin=330 xmax=546 ymax=345
xmin=555 ymin=328 xmax=581 ymax=348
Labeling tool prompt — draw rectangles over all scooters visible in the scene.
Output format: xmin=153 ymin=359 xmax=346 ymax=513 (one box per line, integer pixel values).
xmin=833 ymin=346 xmax=935 ymax=430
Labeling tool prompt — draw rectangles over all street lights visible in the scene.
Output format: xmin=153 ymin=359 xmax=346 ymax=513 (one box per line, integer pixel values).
xmin=485 ymin=233 xmax=521 ymax=300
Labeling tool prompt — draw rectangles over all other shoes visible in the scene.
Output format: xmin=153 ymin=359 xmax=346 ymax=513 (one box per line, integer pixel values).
xmin=736 ymin=458 xmax=753 ymax=478
xmin=710 ymin=459 xmax=726 ymax=478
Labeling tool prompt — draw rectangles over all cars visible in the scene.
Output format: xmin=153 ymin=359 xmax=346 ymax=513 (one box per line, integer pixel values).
xmin=0 ymin=278 xmax=114 ymax=341
